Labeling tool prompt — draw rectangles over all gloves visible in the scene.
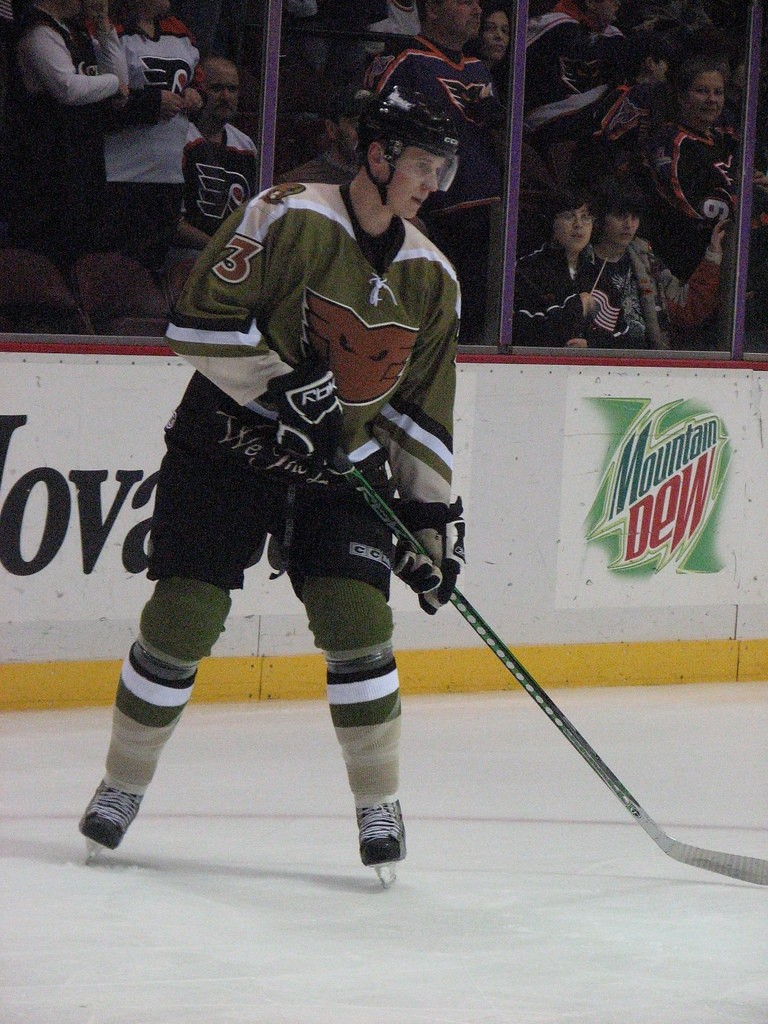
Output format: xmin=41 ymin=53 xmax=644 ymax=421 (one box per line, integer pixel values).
xmin=385 ymin=494 xmax=466 ymax=616
xmin=263 ymin=355 xmax=346 ymax=471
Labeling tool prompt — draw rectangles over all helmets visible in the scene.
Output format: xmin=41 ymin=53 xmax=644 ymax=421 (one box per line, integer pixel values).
xmin=359 ymin=83 xmax=468 ymax=192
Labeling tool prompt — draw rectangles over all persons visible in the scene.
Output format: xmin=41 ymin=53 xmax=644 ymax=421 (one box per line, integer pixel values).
xmin=79 ymin=85 xmax=466 ymax=867
xmin=0 ymin=0 xmax=768 ymax=348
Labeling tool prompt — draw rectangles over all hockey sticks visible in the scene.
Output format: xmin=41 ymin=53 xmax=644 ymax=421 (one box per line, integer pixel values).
xmin=331 ymin=445 xmax=768 ymax=889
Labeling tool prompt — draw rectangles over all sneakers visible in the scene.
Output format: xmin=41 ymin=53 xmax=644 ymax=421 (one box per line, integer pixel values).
xmin=355 ymin=799 xmax=407 ymax=889
xmin=78 ymin=779 xmax=143 ymax=864
